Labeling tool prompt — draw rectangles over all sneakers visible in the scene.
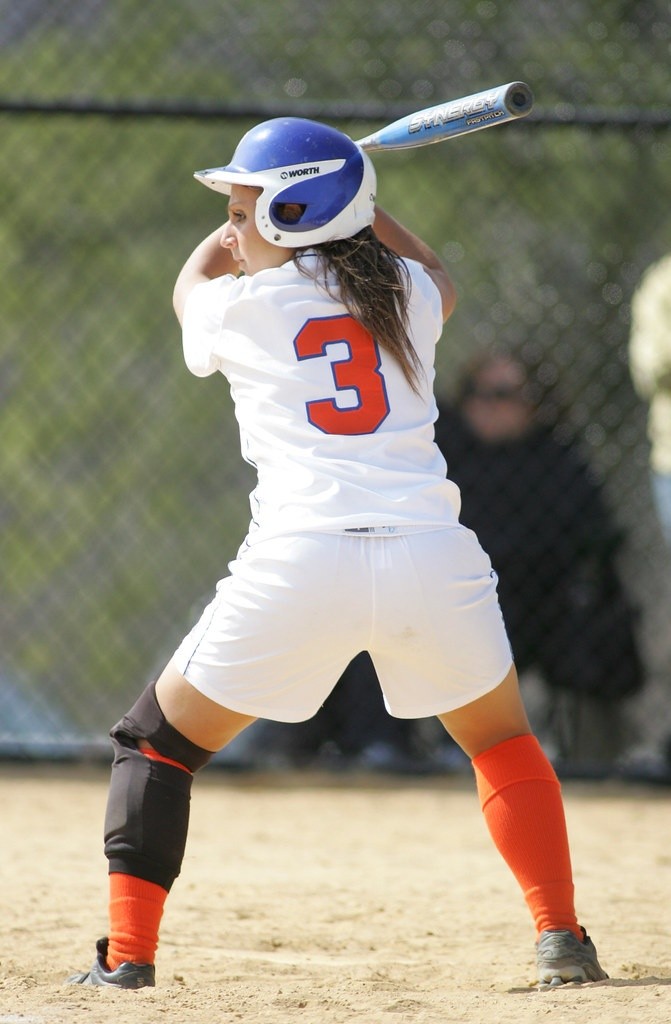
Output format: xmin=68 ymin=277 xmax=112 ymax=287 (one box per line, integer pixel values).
xmin=536 ymin=925 xmax=609 ymax=986
xmin=64 ymin=937 xmax=156 ymax=990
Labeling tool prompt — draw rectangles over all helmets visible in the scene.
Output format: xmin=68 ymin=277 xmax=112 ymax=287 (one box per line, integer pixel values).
xmin=193 ymin=116 xmax=378 ymax=248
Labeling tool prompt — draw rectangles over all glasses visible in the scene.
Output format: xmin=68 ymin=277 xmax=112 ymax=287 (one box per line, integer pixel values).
xmin=466 ymin=379 xmax=524 ymax=404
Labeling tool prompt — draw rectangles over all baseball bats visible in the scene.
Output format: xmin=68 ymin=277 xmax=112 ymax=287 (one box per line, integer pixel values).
xmin=351 ymin=76 xmax=539 ymax=155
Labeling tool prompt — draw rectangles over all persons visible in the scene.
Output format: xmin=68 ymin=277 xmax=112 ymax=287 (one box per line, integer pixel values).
xmin=614 ymin=252 xmax=671 ymax=787
xmin=212 ymin=338 xmax=648 ymax=776
xmin=71 ymin=116 xmax=609 ymax=994
